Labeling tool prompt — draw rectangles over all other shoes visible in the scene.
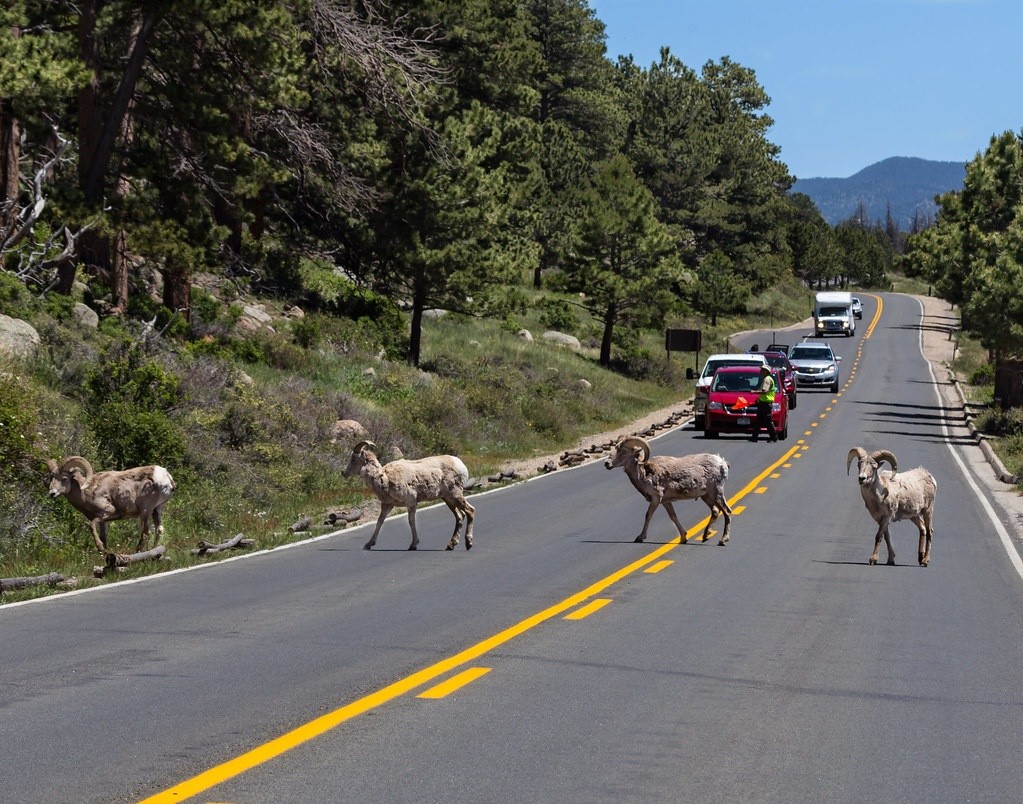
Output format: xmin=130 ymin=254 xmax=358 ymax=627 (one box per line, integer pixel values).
xmin=767 ymin=437 xmax=779 ymax=443
xmin=747 ymin=436 xmax=758 ymax=443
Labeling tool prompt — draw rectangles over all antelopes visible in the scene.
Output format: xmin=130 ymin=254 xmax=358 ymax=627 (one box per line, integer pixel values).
xmin=604 ymin=435 xmax=732 ymax=546
xmin=847 ymin=447 xmax=938 ymax=566
xmin=341 ymin=441 xmax=475 ymax=551
xmin=44 ymin=456 xmax=177 ymax=554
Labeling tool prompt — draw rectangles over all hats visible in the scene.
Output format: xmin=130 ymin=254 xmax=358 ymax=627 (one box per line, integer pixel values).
xmin=759 ymin=364 xmax=772 ymax=374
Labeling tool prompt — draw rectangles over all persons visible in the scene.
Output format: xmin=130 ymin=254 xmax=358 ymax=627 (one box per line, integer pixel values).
xmin=772 ymin=359 xmax=786 ymax=371
xmin=748 ymin=365 xmax=777 ymax=443
xmin=707 ymin=364 xmax=719 ymax=376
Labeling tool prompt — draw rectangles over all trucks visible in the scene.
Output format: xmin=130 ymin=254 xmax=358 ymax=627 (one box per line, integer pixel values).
xmin=812 ymin=290 xmax=856 ymax=338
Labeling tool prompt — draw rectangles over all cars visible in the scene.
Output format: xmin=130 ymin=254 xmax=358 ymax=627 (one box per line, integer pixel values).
xmin=744 ymin=351 xmax=799 ymax=411
xmin=684 ymin=354 xmax=771 ymax=431
xmin=704 ymin=366 xmax=789 ymax=441
xmin=766 ymin=342 xmax=844 ymax=394
xmin=852 ymin=297 xmax=864 ymax=320
